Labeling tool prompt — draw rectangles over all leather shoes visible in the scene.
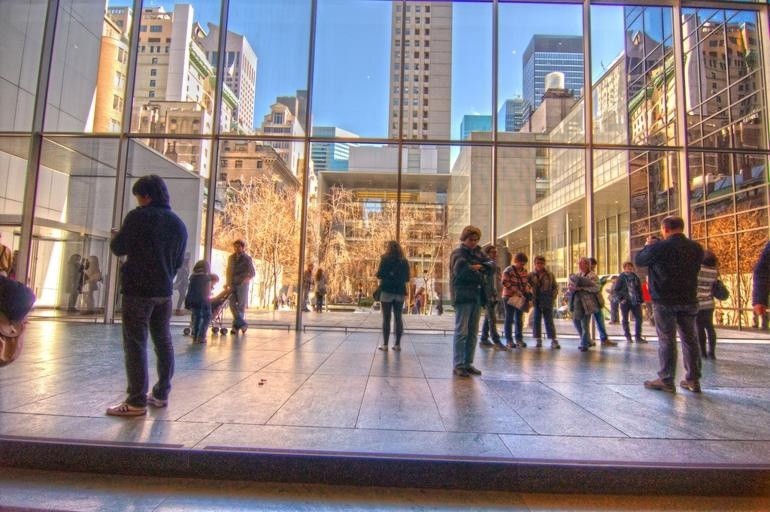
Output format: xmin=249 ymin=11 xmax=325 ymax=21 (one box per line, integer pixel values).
xmin=391 ymin=344 xmax=401 ymax=351
xmin=467 ymin=365 xmax=481 ymax=375
xmin=644 ymin=377 xmax=676 ymax=392
xmin=453 ymin=367 xmax=470 ymax=377
xmin=679 ymin=380 xmax=701 ymax=393
xmin=379 ymin=344 xmax=388 ymax=351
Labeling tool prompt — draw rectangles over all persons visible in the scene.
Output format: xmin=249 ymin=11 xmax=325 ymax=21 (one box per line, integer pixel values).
xmin=449 ymin=226 xmax=496 ymax=377
xmin=479 ymin=244 xmax=730 ymax=361
xmin=410 ymin=295 xmax=443 ymax=315
xmin=373 ymin=240 xmax=410 ymax=351
xmin=106 ymin=173 xmax=187 ymax=417
xmin=0 ymin=244 xmax=19 ymax=281
xmin=62 ymin=251 xmax=103 ymax=318
xmin=752 ymin=241 xmax=770 ymax=317
xmin=274 ymin=262 xmax=332 ymax=315
xmin=635 ymin=216 xmax=704 ymax=393
xmin=173 ymin=239 xmax=256 ymax=344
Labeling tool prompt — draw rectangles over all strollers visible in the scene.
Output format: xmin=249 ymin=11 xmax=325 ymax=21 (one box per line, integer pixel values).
xmin=184 ymin=285 xmax=230 ymax=336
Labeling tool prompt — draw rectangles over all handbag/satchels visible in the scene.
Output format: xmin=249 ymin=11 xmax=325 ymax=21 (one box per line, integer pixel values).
xmin=714 ymin=274 xmax=730 ymax=300
xmin=0 ymin=311 xmax=29 ymax=368
xmin=507 ymin=265 xmax=530 ymax=313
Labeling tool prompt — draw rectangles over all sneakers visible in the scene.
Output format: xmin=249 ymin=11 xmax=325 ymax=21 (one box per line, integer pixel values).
xmin=199 ymin=337 xmax=207 ymax=343
xmin=192 ymin=337 xmax=200 ymax=343
xmin=147 ymin=392 xmax=169 ymax=408
xmin=240 ymin=322 xmax=248 ymax=333
xmin=479 ymin=338 xmax=647 ymax=351
xmin=230 ymin=327 xmax=236 ymax=334
xmin=106 ymin=401 xmax=147 ymax=417
